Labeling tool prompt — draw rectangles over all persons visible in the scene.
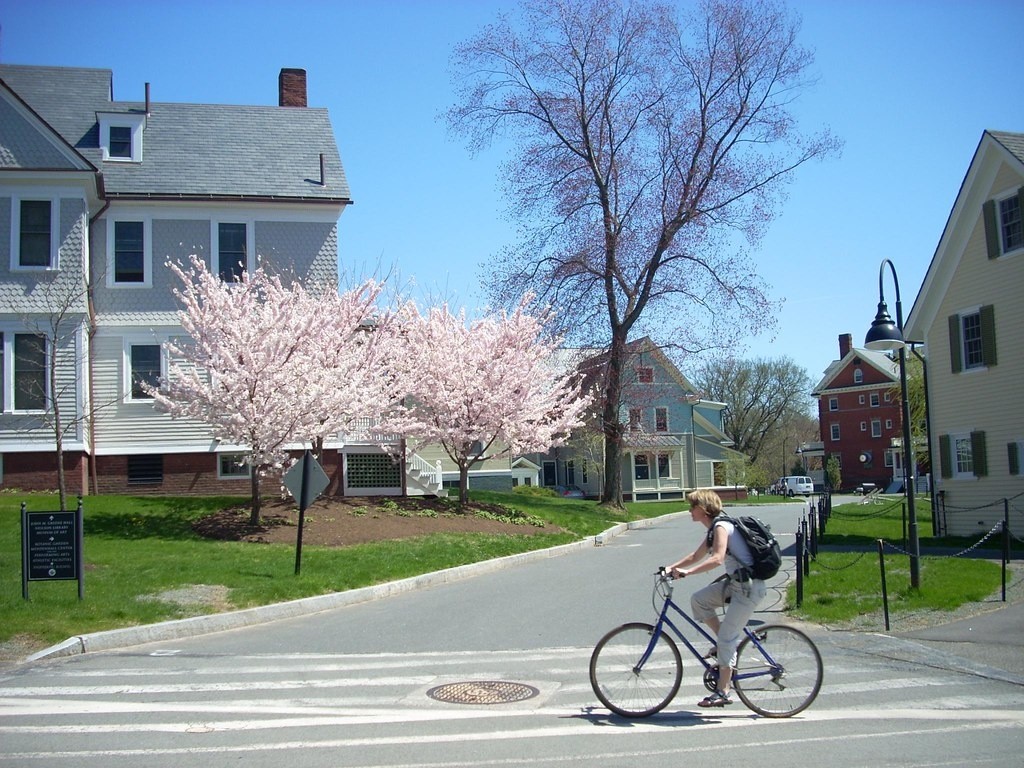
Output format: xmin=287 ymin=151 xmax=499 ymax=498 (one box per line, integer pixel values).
xmin=661 ymin=490 xmax=766 ymax=707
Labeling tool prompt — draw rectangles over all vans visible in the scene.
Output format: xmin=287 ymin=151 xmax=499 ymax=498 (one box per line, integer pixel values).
xmin=765 ymin=476 xmax=814 ymax=497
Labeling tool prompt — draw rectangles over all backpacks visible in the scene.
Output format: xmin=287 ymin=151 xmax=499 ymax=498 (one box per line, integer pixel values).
xmin=706 ymin=510 xmax=782 ymax=583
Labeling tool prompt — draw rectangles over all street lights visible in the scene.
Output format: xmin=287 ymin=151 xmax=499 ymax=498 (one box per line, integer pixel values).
xmin=864 ymin=258 xmax=919 ymax=591
xmin=783 ymin=437 xmax=803 ymax=499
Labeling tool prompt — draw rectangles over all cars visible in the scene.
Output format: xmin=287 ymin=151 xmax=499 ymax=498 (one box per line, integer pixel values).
xmin=553 ymin=485 xmax=586 ymax=500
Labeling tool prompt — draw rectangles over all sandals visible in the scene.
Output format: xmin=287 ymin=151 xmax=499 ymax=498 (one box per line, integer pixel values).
xmin=698 ymin=690 xmax=733 ymax=706
xmin=705 ymin=646 xmax=717 ymax=657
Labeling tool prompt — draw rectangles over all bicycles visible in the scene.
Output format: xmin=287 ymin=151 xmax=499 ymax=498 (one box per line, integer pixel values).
xmin=590 ymin=565 xmax=823 ymax=719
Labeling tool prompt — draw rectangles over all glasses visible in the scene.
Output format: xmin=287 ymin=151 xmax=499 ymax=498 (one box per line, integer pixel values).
xmin=691 ymin=501 xmax=700 ymax=509
